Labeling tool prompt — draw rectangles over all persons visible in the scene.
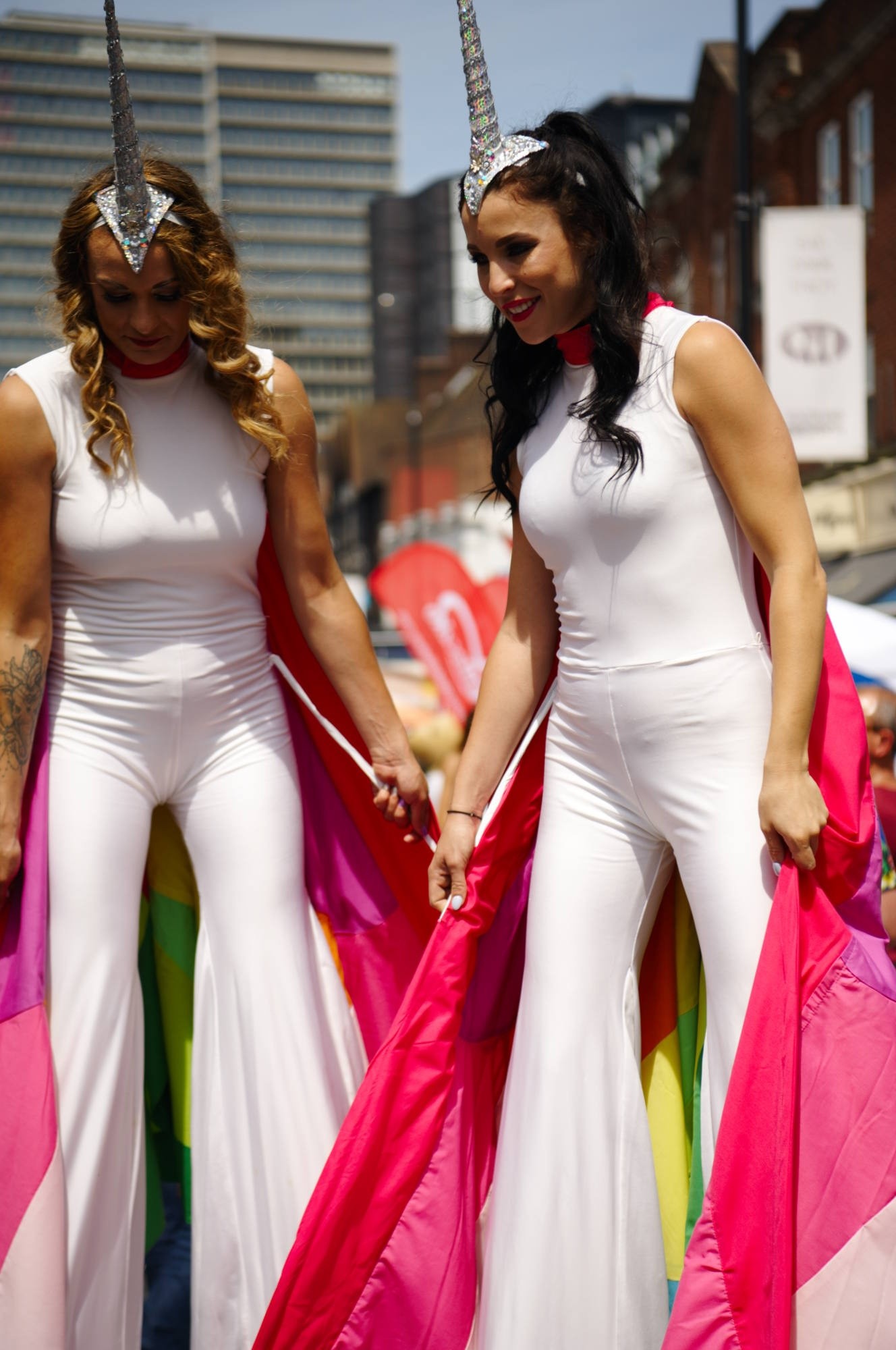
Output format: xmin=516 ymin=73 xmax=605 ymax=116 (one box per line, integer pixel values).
xmin=852 ymin=683 xmax=895 ymax=861
xmin=429 ymin=111 xmax=896 ymax=1350
xmin=0 ymin=144 xmax=431 ymax=1350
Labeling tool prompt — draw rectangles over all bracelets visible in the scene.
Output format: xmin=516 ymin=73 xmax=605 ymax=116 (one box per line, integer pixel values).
xmin=448 ymin=810 xmax=482 ymax=820
